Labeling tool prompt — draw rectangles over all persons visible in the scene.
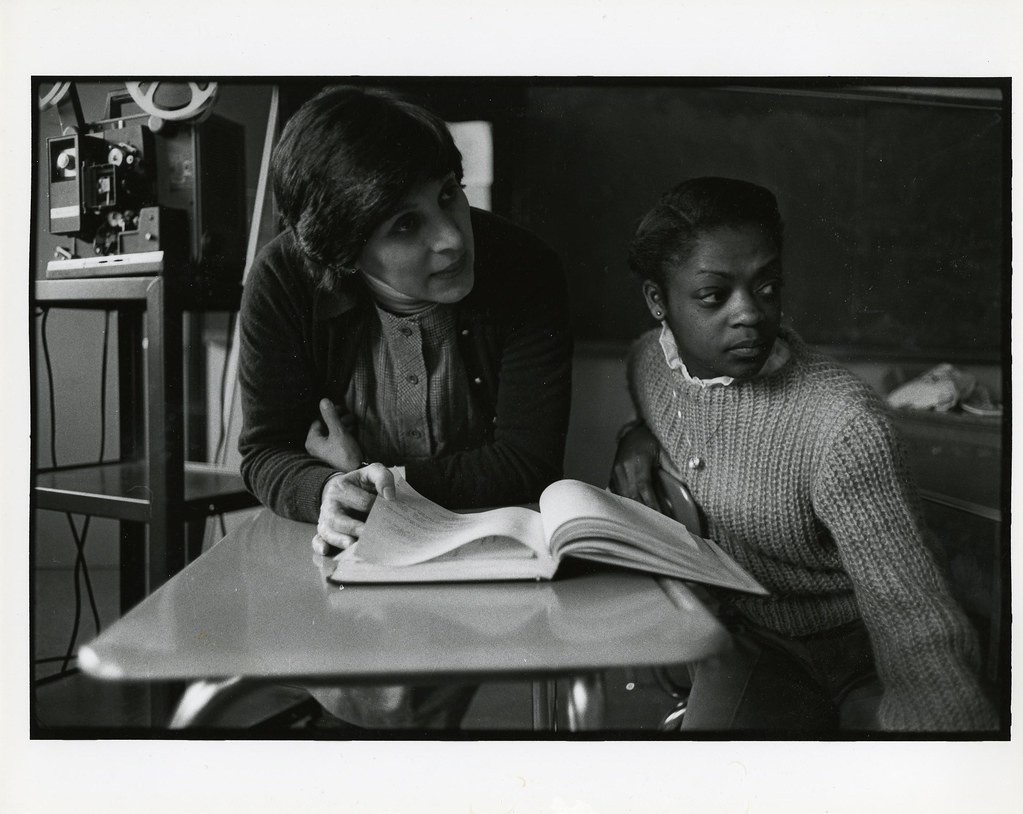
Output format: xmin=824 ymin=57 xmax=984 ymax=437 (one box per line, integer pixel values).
xmin=237 ymin=84 xmax=574 ymax=556
xmin=609 ymin=177 xmax=1001 ymax=732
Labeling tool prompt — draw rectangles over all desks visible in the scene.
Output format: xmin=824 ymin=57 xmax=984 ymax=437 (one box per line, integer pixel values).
xmin=74 ymin=501 xmax=735 ymax=740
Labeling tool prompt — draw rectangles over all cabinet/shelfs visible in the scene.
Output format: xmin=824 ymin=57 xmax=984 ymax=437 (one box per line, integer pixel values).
xmin=30 ymin=275 xmax=262 ymax=740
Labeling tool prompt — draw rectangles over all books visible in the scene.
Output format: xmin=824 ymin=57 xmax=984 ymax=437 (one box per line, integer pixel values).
xmin=324 ymin=466 xmax=770 ymax=597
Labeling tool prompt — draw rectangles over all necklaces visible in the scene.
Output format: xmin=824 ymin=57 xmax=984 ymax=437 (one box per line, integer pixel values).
xmin=670 ymin=370 xmax=745 ymax=470
